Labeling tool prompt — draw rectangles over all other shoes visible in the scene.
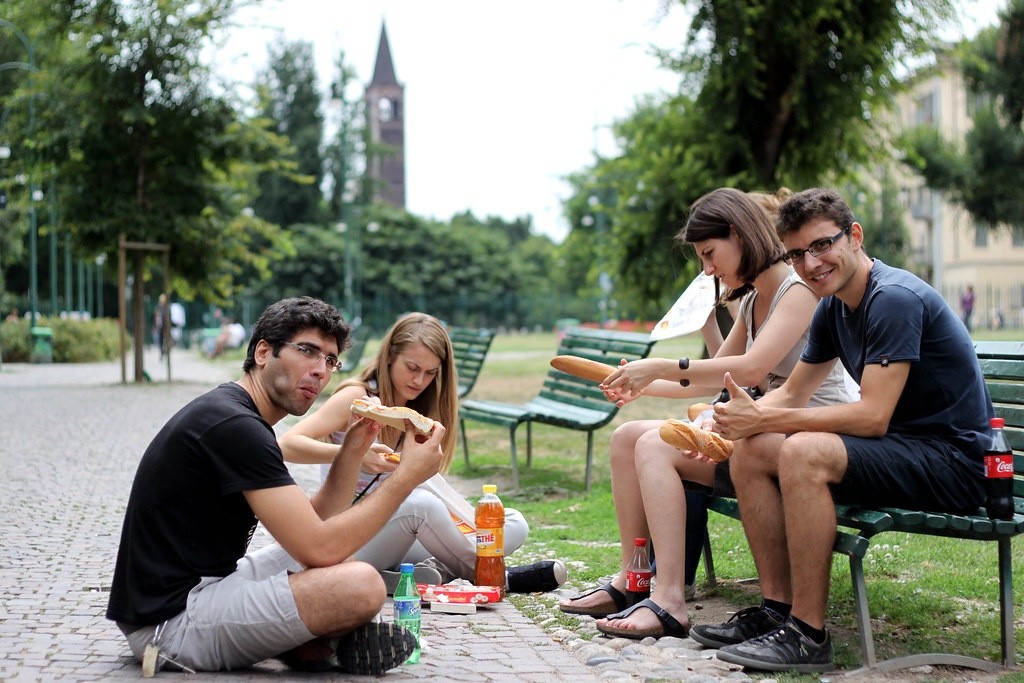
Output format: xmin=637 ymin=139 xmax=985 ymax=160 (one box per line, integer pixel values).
xmin=596 ymin=571 xmax=621 ymax=589
xmin=650 ymin=576 xmax=697 ymax=601
xmin=282 ymin=623 xmax=417 ymax=676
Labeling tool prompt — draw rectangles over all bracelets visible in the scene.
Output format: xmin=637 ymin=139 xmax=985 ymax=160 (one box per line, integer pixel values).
xmin=679 ymin=357 xmax=690 ymax=387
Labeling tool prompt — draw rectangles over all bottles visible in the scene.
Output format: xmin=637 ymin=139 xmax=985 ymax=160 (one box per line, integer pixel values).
xmin=983 ymin=417 xmax=1015 ymax=521
xmin=392 ymin=563 xmax=421 ymax=664
xmin=473 ymin=484 xmax=506 ymax=602
xmin=624 ymin=537 xmax=651 ymax=607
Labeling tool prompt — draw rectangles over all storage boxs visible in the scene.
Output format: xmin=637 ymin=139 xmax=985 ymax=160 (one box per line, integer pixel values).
xmin=417 ymin=582 xmax=500 ymax=614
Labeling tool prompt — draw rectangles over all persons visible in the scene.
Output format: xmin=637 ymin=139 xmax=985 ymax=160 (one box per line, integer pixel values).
xmin=675 ymin=188 xmax=997 ymax=672
xmin=595 ymin=187 xmax=794 ymax=601
xmin=960 ymin=286 xmax=974 ymax=332
xmin=560 ymin=187 xmax=861 ymax=641
xmin=206 ymin=314 xmax=246 ymax=359
xmin=155 ymin=293 xmax=176 ymax=360
xmin=105 ymin=295 xmax=446 ymax=676
xmin=276 ymin=312 xmax=567 ymax=594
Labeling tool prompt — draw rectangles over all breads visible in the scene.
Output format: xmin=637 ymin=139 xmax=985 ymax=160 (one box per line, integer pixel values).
xmin=384 ymin=452 xmax=400 ymax=463
xmin=658 ymin=418 xmax=735 ymax=461
xmin=351 ymin=399 xmax=435 ymax=435
xmin=688 ymin=403 xmax=714 ymax=421
xmin=549 ymin=355 xmax=619 ymax=383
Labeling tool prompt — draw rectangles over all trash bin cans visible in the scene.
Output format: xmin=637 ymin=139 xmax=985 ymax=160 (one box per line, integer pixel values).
xmin=30 ymin=326 xmax=53 ymax=364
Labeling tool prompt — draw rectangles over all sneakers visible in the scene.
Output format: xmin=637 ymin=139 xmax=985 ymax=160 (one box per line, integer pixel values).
xmin=503 ymin=559 xmax=567 ymax=594
xmin=714 ymin=616 xmax=834 ymax=673
xmin=380 ymin=566 xmax=444 ymax=596
xmin=688 ymin=603 xmax=787 ymax=647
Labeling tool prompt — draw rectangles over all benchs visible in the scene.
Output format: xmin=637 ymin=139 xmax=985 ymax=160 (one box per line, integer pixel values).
xmin=331 ymin=328 xmax=370 ymax=378
xmin=458 ymin=336 xmax=659 ymax=489
xmin=447 ymin=329 xmax=498 ymax=468
xmin=699 ymin=341 xmax=1024 ymax=682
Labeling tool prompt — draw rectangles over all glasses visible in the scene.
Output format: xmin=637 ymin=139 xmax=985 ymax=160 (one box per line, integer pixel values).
xmin=278 ymin=339 xmax=343 ymax=373
xmin=782 ymin=226 xmax=849 ymax=266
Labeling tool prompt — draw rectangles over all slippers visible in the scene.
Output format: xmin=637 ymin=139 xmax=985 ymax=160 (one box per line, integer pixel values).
xmin=595 ymin=599 xmax=690 ymax=638
xmin=559 ymin=582 xmax=635 ymax=620
